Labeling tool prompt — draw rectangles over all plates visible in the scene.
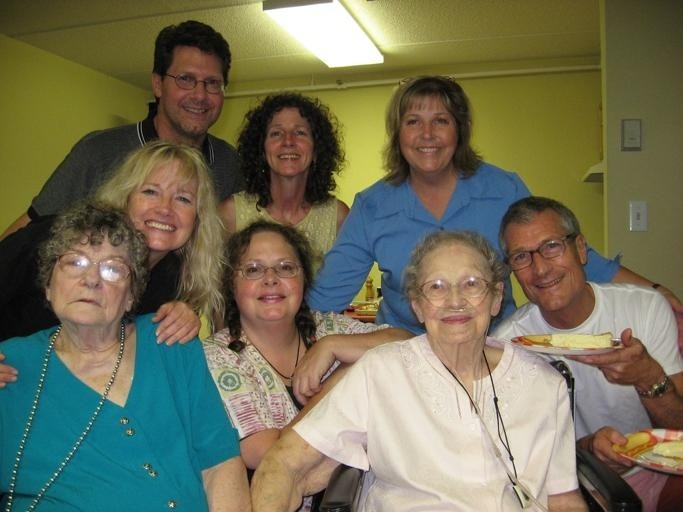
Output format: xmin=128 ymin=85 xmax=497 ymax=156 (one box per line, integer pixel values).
xmin=355 ymin=306 xmax=380 ymax=316
xmin=614 ymin=429 xmax=683 ymax=479
xmin=510 ymin=333 xmax=624 ymax=356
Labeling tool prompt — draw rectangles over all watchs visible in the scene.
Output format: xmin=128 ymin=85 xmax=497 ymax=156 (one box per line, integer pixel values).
xmin=638 ymin=376 xmax=670 ymax=399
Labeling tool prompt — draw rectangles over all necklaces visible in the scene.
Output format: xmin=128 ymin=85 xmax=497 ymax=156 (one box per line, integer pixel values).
xmin=4 ymin=323 xmax=127 ymax=511
xmin=247 ymin=333 xmax=302 ymax=379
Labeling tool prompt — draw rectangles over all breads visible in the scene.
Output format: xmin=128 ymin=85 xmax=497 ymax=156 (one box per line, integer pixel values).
xmin=551 ymin=333 xmax=613 ymax=349
xmin=652 ymin=441 xmax=682 ymax=460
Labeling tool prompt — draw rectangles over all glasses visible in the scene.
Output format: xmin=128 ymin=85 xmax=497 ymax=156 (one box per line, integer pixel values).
xmin=504 ymin=232 xmax=578 ymax=271
xmin=234 ymin=261 xmax=304 ymax=281
xmin=417 ymin=277 xmax=496 ymax=300
xmin=163 ymin=72 xmax=225 ymax=94
xmin=67 ymin=253 xmax=134 ymax=293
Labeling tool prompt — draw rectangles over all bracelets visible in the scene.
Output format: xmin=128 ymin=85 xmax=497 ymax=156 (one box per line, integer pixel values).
xmin=653 ymin=283 xmax=659 ymax=291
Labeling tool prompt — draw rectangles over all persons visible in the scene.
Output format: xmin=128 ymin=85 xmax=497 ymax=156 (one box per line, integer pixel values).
xmin=0 ymin=143 xmax=230 ymax=392
xmin=250 ymin=231 xmax=589 ymax=511
xmin=197 ymin=222 xmax=418 ymax=511
xmin=212 ymin=93 xmax=352 ymax=331
xmin=303 ymin=73 xmax=683 ymax=340
xmin=0 ymin=201 xmax=252 ymax=512
xmin=0 ymin=20 xmax=235 ymax=242
xmin=488 ymin=196 xmax=682 ymax=512
